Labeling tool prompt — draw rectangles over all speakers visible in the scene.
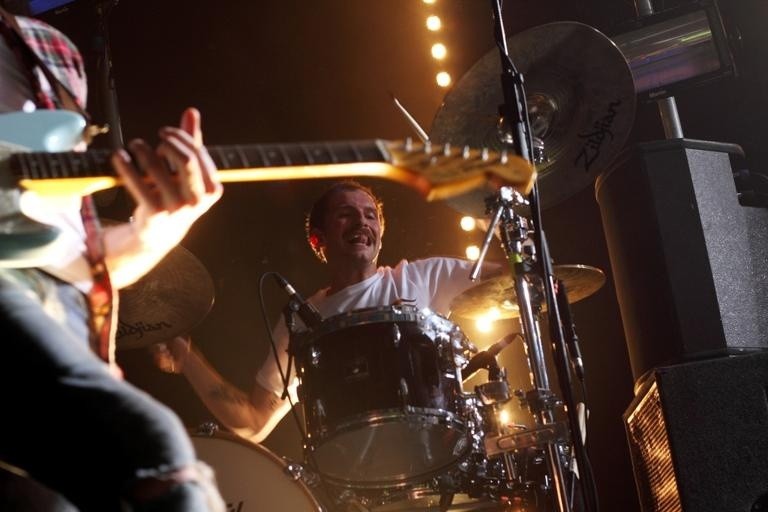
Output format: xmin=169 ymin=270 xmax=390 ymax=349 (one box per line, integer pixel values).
xmin=592 ymin=139 xmax=768 ymax=399
xmin=621 ymin=350 xmax=768 ymax=511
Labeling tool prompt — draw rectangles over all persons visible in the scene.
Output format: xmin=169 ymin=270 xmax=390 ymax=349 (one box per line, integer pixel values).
xmin=150 ymin=181 xmax=505 ymax=445
xmin=1 ymin=11 xmax=227 ymax=512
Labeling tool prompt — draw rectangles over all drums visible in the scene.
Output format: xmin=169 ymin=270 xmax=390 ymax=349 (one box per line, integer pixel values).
xmin=185 ymin=424 xmax=327 ymax=511
xmin=300 ymin=306 xmax=473 ymax=489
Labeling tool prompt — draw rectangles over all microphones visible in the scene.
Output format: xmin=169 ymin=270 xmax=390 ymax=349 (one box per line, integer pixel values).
xmin=284 ymin=279 xmax=319 ymax=330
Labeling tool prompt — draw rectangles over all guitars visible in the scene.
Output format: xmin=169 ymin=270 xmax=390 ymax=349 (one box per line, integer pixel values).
xmin=0 ymin=138 xmax=538 ymax=261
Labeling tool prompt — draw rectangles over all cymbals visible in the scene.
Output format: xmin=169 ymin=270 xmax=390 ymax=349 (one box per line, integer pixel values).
xmin=102 ymin=217 xmax=217 ymax=350
xmin=431 ymin=21 xmax=636 ymax=218
xmin=450 ymin=264 xmax=605 ymax=320
